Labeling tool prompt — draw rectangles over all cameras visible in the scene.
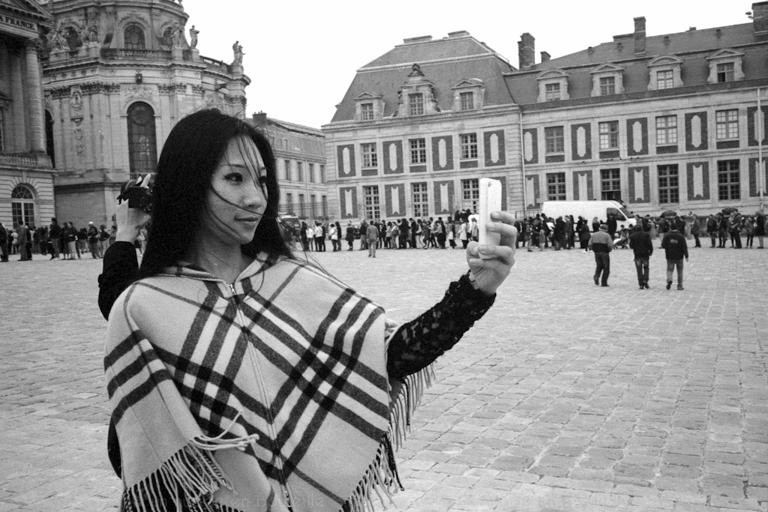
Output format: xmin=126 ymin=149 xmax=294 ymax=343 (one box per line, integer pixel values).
xmin=117 ymin=172 xmax=155 ymax=213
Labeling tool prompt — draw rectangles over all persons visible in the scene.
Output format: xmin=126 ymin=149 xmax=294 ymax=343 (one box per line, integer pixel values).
xmin=515 ymin=212 xmax=767 ymax=252
xmin=0 ymin=217 xmax=149 ymax=261
xmin=232 ymin=40 xmax=243 ymax=65
xmin=97 ymin=173 xmax=155 ymax=479
xmin=101 ymin=109 xmax=518 ymax=512
xmin=279 ymin=208 xmax=478 ymax=257
xmin=588 ymin=223 xmax=613 ymax=286
xmin=661 ymin=223 xmax=689 ymax=290
xmin=189 ymin=25 xmax=201 ymax=47
xmin=630 ymin=225 xmax=653 ymax=289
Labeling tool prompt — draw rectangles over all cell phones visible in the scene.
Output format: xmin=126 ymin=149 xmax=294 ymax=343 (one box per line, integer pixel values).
xmin=479 ymin=177 xmax=502 ymax=260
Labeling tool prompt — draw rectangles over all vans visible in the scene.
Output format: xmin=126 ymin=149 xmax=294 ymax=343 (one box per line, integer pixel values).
xmin=542 ymin=200 xmax=637 ymax=238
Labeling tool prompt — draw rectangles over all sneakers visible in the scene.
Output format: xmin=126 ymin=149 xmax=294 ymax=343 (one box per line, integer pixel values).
xmin=677 ymin=288 xmax=684 ymax=290
xmin=666 ymin=281 xmax=673 ymax=289
xmin=640 ymin=280 xmax=649 ymax=289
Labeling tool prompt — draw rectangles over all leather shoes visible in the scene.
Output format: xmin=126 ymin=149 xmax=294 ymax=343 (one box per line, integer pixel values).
xmin=594 ymin=276 xmax=599 ymax=285
xmin=601 ymin=284 xmax=610 ymax=286
